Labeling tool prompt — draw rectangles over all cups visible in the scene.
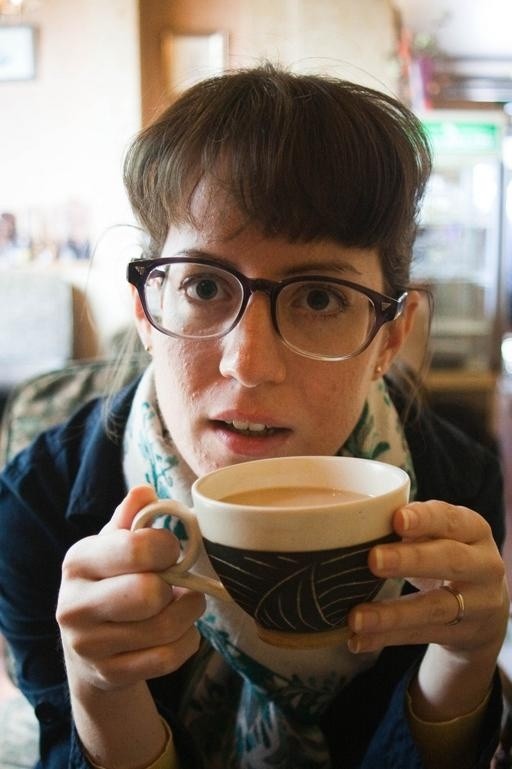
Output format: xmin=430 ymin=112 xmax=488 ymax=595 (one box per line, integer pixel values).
xmin=130 ymin=455 xmax=411 ymax=649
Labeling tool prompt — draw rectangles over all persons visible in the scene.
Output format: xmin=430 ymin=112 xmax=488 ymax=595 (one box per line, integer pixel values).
xmin=0 ymin=64 xmax=512 ymax=769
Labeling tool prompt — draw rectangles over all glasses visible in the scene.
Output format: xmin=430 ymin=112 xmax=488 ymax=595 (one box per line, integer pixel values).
xmin=122 ymin=254 xmax=410 ymax=363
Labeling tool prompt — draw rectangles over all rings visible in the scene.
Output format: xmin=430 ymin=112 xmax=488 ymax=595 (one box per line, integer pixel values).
xmin=436 ymin=583 xmax=467 ymax=626
